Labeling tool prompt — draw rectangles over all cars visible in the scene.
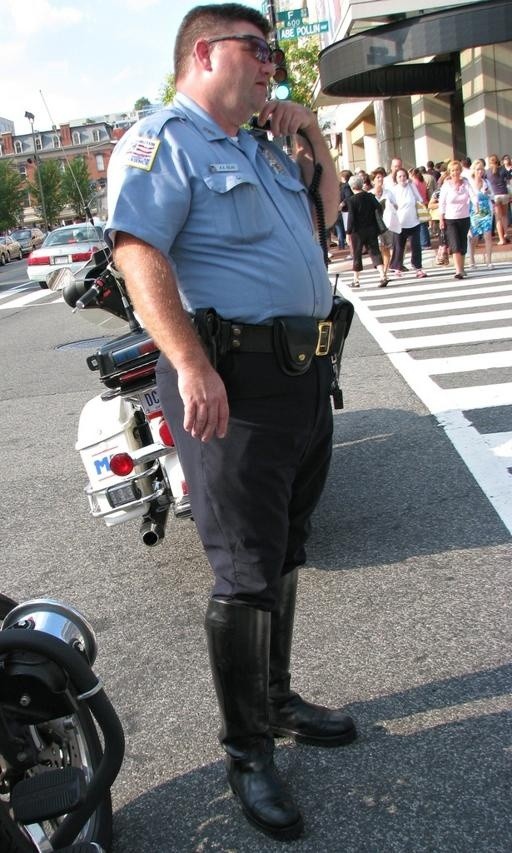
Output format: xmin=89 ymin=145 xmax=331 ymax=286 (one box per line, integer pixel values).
xmin=27 ymin=219 xmax=108 ymax=290
xmin=11 ymin=227 xmax=47 ymax=255
xmin=0 ymin=236 xmax=23 ymax=265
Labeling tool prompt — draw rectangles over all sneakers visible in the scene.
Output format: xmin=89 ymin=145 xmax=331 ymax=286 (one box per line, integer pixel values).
xmin=455 ymin=264 xmax=494 ymax=279
xmin=389 ymin=264 xmax=408 ymax=277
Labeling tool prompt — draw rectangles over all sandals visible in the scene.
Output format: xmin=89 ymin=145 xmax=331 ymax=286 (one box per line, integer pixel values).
xmin=416 ymin=270 xmax=426 ymax=278
xmin=378 ymin=279 xmax=388 ymax=287
xmin=346 ymin=282 xmax=360 ymax=287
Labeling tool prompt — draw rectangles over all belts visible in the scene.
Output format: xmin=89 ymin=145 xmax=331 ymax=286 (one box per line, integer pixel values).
xmin=231 ymin=320 xmax=334 ymax=356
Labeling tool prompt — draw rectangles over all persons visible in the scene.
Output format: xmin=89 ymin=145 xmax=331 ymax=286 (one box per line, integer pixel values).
xmin=326 ymin=154 xmax=512 ymax=286
xmin=73 ymin=216 xmax=89 ymax=240
xmin=101 ymin=1 xmax=353 ymax=843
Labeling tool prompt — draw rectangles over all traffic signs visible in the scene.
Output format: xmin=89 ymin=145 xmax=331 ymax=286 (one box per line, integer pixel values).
xmin=280 ymin=21 xmax=329 ymax=40
xmin=277 ymin=8 xmax=309 ymax=21
xmin=258 ymin=1 xmax=270 ymax=15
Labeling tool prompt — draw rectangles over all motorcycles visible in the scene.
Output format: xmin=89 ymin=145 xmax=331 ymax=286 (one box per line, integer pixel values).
xmin=52 ymin=247 xmax=198 ymax=546
xmin=0 ymin=592 xmax=126 ymax=853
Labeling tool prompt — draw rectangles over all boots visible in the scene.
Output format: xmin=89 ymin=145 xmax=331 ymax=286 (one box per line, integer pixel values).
xmin=269 ymin=567 xmax=356 ymax=747
xmin=206 ymin=599 xmax=303 ymax=841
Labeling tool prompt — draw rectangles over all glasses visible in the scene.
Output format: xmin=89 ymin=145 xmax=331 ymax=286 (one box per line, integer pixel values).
xmin=193 ymin=35 xmax=284 ymax=65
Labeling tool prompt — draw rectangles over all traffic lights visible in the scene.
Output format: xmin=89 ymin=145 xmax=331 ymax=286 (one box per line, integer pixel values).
xmin=271 ymin=49 xmax=290 ymax=100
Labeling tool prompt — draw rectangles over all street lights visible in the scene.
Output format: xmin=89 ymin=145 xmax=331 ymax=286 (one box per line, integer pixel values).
xmin=23 ymin=110 xmax=49 ymax=229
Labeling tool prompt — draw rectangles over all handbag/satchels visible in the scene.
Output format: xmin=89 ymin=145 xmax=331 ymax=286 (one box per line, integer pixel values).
xmin=429 ymin=208 xmax=439 ymax=221
xmin=416 ymin=201 xmax=432 ymax=224
xmin=375 ymin=209 xmax=388 ymax=235
xmin=436 ymin=245 xmax=449 ymax=265
